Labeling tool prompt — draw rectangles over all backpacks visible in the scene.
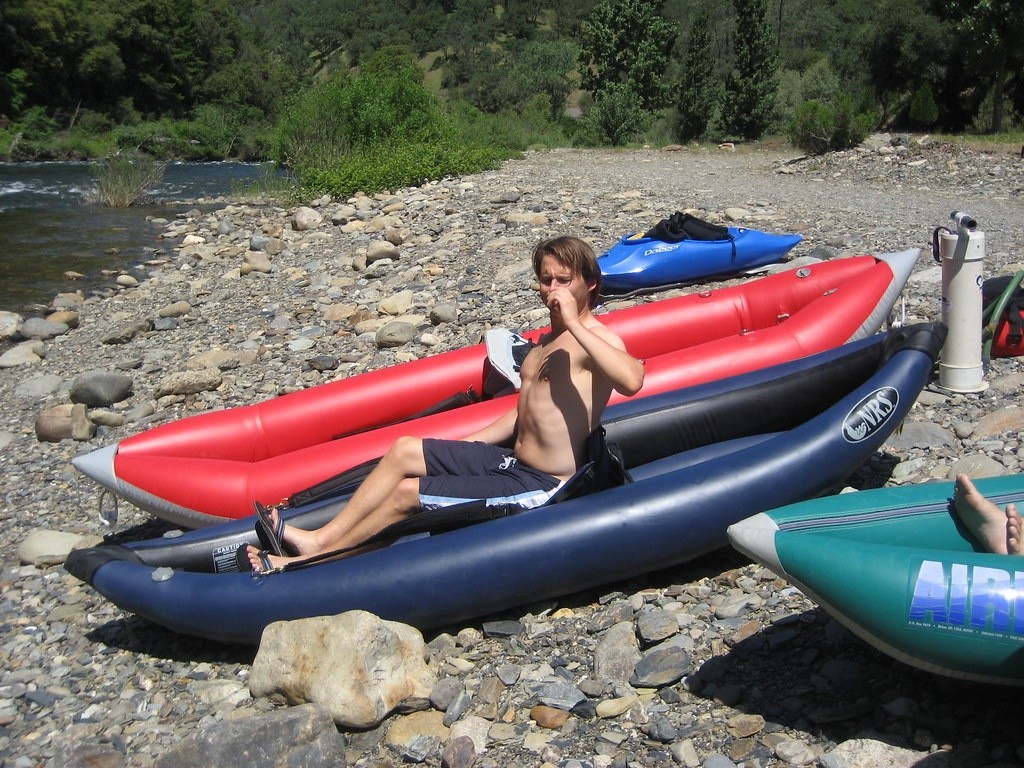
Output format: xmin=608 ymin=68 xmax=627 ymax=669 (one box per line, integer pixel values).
xmin=983 ymin=276 xmax=1024 ymax=357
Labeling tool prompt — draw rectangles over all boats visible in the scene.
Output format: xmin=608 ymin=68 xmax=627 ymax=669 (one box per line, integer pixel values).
xmin=71 ymin=246 xmax=922 ymax=530
xmin=726 ymin=472 xmax=1024 ymax=691
xmin=594 ymin=224 xmax=803 ymax=294
xmin=60 ymin=319 xmax=950 ymax=649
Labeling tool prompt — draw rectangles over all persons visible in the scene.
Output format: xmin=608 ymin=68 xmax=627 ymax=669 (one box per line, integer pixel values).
xmin=954 ymin=472 xmax=1024 ymax=554
xmin=237 ymin=236 xmax=644 ymax=573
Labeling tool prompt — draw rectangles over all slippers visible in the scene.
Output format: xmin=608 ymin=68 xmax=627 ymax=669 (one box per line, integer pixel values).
xmin=254 ymin=500 xmax=285 ymax=558
xmin=235 ymin=542 xmax=274 ymax=572
